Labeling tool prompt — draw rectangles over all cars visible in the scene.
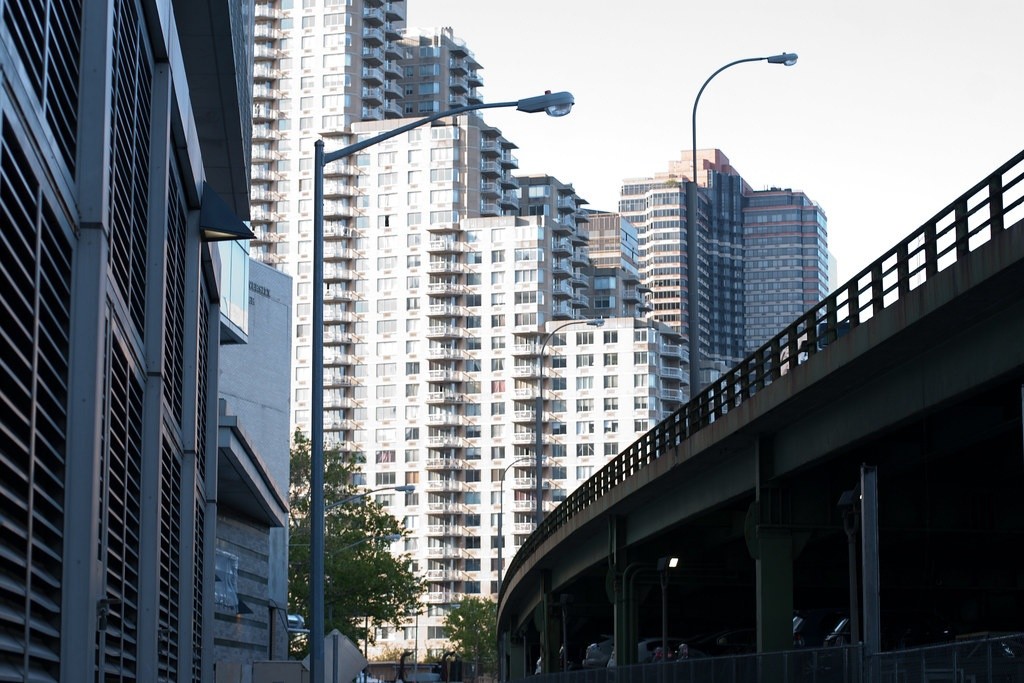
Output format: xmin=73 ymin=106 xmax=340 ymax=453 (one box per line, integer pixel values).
xmin=689 ymin=628 xmax=758 ymax=660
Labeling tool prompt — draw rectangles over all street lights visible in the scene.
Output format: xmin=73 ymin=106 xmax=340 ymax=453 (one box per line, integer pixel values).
xmin=692 ymin=47 xmax=798 ymax=394
xmin=536 ymin=318 xmax=607 ymax=521
xmin=320 ymin=483 xmax=415 ymax=683
xmin=657 ymin=556 xmax=681 ymax=682
xmin=311 ymin=87 xmax=577 ymax=683
xmin=499 ymin=455 xmax=548 ymax=590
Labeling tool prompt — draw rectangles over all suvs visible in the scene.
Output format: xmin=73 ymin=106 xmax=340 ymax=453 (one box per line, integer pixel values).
xmin=533 ymin=656 xmax=543 ymax=675
xmin=582 ymin=633 xmax=614 ymax=669
xmin=558 ymin=641 xmax=582 ymax=672
xmin=793 ymin=607 xmax=851 ymax=677
xmin=823 ymin=612 xmax=955 ymax=683
xmin=609 ymin=638 xmax=709 ymax=676
